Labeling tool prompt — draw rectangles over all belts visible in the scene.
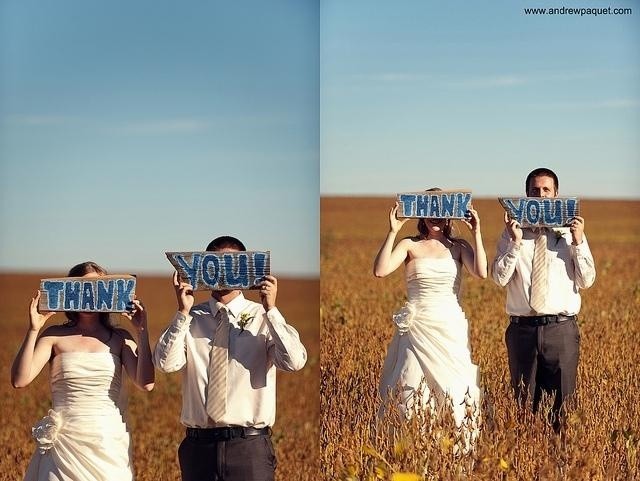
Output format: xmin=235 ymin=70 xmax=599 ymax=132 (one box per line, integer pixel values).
xmin=509 ymin=310 xmax=579 ymax=327
xmin=185 ymin=422 xmax=272 ymax=441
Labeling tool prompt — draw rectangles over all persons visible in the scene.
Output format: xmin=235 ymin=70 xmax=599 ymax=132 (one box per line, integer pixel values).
xmin=373 ymin=168 xmax=595 ymax=468
xmin=11 ymin=237 xmax=308 ymax=481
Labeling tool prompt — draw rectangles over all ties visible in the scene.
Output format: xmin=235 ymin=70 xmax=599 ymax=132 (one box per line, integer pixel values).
xmin=530 ymin=226 xmax=548 ymax=316
xmin=205 ymin=306 xmax=231 ymax=423
